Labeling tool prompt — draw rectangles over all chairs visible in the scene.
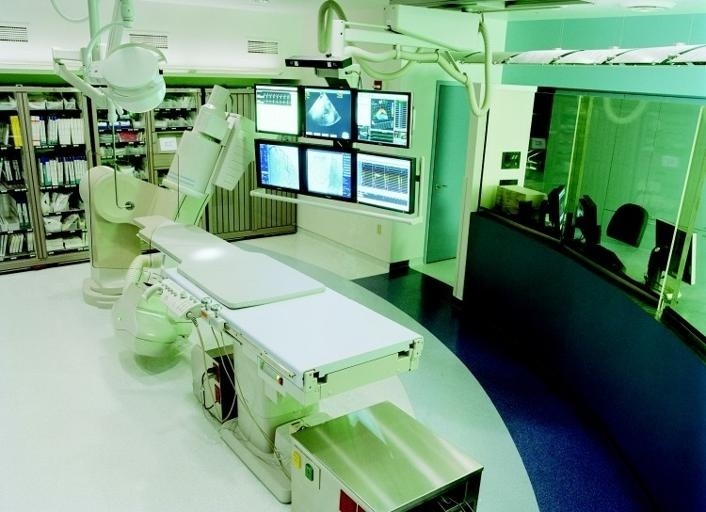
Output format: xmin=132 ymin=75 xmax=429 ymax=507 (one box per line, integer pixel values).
xmin=606 ymin=202 xmax=649 ymax=248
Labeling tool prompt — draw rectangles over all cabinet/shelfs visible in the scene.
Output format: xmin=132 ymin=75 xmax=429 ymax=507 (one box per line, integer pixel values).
xmin=90 ymin=87 xmax=202 ymax=188
xmin=204 ymin=88 xmax=297 ymax=241
xmin=0 ymin=86 xmax=94 ymax=274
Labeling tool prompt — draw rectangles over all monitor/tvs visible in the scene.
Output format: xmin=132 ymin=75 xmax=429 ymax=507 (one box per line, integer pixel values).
xmin=254 ymin=84 xmax=301 ymax=135
xmin=302 ymin=86 xmax=353 ymax=141
xmin=355 ymin=151 xmax=416 ymax=214
xmin=576 ymin=195 xmax=601 ymax=244
xmin=303 ymin=143 xmax=355 ymax=203
xmin=656 ymin=219 xmax=697 ymax=286
xmin=548 ymin=185 xmax=567 ymax=226
xmin=255 ymin=139 xmax=302 ymax=194
xmin=354 ymin=89 xmax=411 ymax=148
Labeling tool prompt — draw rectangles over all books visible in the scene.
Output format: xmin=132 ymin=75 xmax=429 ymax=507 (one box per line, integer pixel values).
xmin=0 ymin=96 xmax=88 ymax=258
